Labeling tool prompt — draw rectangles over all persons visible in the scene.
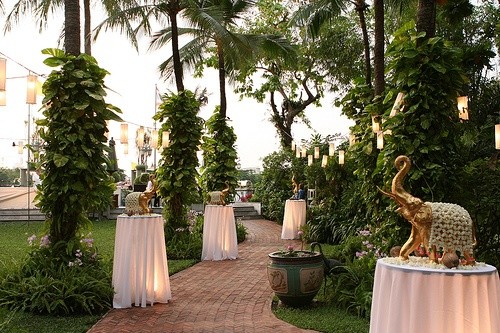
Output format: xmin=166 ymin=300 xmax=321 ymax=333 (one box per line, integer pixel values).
xmin=146 ymin=174 xmax=161 ymax=207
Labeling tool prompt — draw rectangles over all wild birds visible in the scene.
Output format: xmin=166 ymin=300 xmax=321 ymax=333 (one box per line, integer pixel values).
xmin=311 ymin=241 xmax=348 ymax=295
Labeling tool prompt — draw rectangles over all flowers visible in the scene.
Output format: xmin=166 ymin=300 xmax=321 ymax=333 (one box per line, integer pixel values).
xmin=282 ymin=224 xmax=308 ymax=257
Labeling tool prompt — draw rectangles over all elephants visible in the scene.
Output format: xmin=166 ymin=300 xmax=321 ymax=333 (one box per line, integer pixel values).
xmin=375 ymin=155 xmax=477 ymax=265
xmin=205 ymin=181 xmax=231 ymax=205
xmin=125 ymin=175 xmax=159 ymax=216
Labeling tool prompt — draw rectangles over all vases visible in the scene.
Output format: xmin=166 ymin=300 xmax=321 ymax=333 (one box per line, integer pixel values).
xmin=267 ymin=249 xmax=324 ymax=306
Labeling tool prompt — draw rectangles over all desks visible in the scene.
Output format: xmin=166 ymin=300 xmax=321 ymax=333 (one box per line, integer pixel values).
xmin=201 ymin=205 xmax=237 ymax=261
xmin=370 ymin=258 xmax=500 ymax=333
xmin=112 ymin=214 xmax=172 ymax=309
xmin=282 ymin=199 xmax=305 ymax=240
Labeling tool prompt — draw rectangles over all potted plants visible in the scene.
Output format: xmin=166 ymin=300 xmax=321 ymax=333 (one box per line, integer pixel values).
xmin=135 ymin=165 xmax=145 ymax=177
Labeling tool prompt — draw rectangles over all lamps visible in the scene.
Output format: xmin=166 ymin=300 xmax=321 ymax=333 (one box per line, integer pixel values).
xmin=26 ymin=69 xmax=38 ymax=103
xmin=339 ymin=150 xmax=344 ymax=164
xmin=292 ymin=141 xmax=327 ymax=167
xmin=372 ymin=116 xmax=392 ymax=149
xmin=121 ymin=124 xmax=128 ymax=144
xmin=391 ymin=92 xmax=407 ymax=117
xmin=329 ymin=143 xmax=334 ymax=156
xmin=152 ymin=129 xmax=158 ymax=149
xmin=0 ymin=58 xmax=6 ymax=106
xmin=349 ymin=135 xmax=356 ymax=147
xmin=137 ymin=128 xmax=144 ymax=148
xmin=163 ymin=131 xmax=170 ymax=148
xmin=457 ymin=96 xmax=469 ymax=122
xmin=494 ymin=124 xmax=500 ymax=150
